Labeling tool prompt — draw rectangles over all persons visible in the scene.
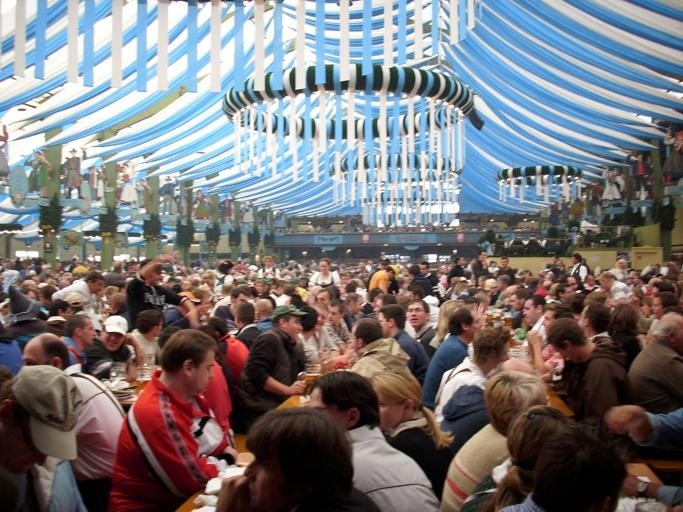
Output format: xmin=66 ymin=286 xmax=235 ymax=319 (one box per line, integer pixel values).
xmin=1 ymin=249 xmax=683 ymax=512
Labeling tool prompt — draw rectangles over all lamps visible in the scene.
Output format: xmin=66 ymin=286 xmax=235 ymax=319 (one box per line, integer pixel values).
xmin=302 ymin=251 xmax=308 ymax=257
xmin=453 ymin=249 xmax=458 ymax=255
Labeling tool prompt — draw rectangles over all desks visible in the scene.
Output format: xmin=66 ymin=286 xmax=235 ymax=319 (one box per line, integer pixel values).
xmin=109 ymin=314 xmax=683 ymax=512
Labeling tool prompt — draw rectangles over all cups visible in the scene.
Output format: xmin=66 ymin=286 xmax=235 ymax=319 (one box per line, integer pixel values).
xmin=110 ymin=362 xmax=153 ymax=395
xmin=484 ymin=313 xmax=513 ymax=329
xmin=306 ymin=374 xmax=321 ymax=393
xmin=634 ymin=502 xmax=667 ymax=512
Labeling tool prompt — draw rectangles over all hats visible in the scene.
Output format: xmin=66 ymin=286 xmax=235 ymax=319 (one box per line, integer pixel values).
xmin=13 ymin=364 xmax=83 ymax=460
xmin=64 ymin=293 xmax=84 ymax=307
xmin=103 ymin=315 xmax=129 ymax=337
xmin=272 ymin=305 xmax=309 ymax=319
xmin=72 ymin=265 xmax=89 ymax=275
xmin=178 ymin=291 xmax=201 ymax=303
xmin=3 ymin=286 xmax=41 ymax=324
xmin=388 ymin=264 xmax=400 ymax=280
